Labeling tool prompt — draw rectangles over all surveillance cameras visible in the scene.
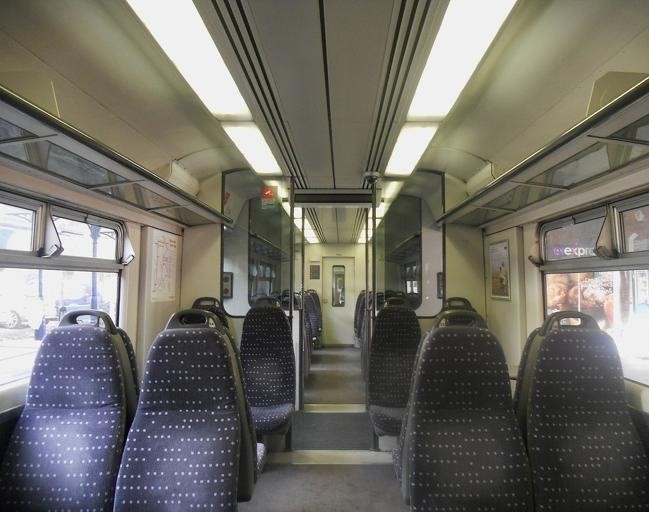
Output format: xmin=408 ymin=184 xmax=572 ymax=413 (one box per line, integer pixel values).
xmin=363 ymin=171 xmax=380 ymax=184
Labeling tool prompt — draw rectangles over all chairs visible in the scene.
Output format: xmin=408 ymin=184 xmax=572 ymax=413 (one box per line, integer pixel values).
xmin=395 ymin=314 xmax=649 ymax=512
xmin=184 ymin=285 xmax=323 ymax=453
xmin=357 ymin=293 xmax=488 ymax=447
xmin=3 ymin=311 xmax=267 ymax=512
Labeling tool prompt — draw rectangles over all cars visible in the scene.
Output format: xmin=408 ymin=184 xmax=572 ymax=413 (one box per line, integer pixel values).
xmin=55 ymin=295 xmax=111 ymax=324
xmin=0 ymin=297 xmax=57 ymax=329
